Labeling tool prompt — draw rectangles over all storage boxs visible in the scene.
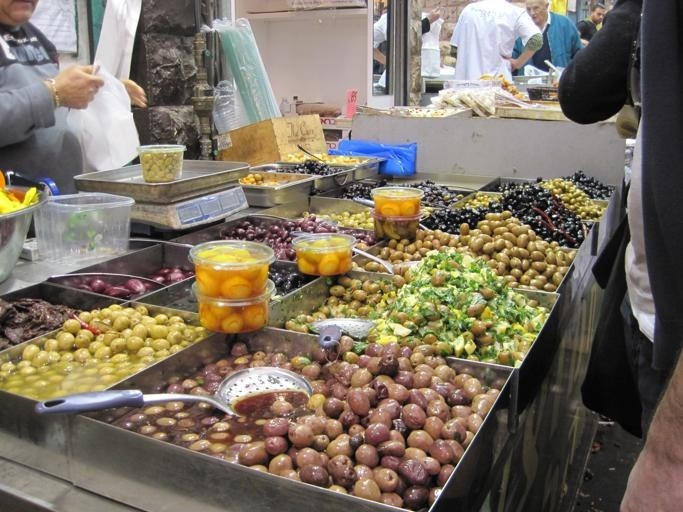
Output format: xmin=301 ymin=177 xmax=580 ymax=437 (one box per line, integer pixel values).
xmin=216 ymin=112 xmax=329 ymax=167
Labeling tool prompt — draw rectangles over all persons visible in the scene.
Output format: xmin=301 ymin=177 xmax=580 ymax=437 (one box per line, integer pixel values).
xmin=449 ymin=1 xmax=543 ymax=86
xmin=557 ymin=1 xmax=683 ymax=510
xmin=372 ymin=1 xmax=440 ymax=95
xmin=511 ymin=1 xmax=583 ymax=79
xmin=574 ymin=4 xmax=605 ymax=41
xmin=0 ymin=1 xmax=150 ymax=196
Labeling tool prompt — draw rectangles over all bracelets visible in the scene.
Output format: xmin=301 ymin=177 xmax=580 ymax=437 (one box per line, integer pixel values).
xmin=47 ymin=77 xmax=60 ymax=108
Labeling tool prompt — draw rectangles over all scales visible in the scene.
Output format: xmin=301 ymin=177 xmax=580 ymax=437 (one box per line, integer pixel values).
xmin=73 ymin=160 xmax=250 ymax=241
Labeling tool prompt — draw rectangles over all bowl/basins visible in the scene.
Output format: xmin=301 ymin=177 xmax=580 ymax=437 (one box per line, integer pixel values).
xmin=0 ymin=184 xmax=49 ymax=286
